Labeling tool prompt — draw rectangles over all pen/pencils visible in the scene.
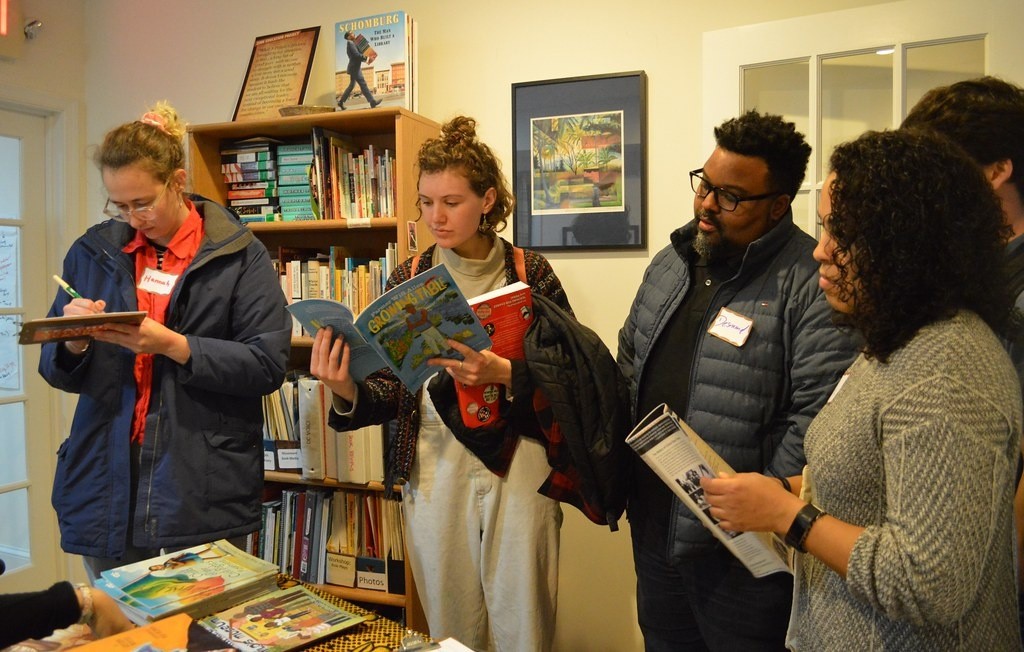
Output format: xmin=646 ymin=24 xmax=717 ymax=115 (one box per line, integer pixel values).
xmin=53 ymin=275 xmax=84 ymax=299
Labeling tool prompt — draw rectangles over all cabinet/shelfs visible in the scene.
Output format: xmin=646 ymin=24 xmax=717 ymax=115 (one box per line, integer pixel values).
xmin=187 ymin=107 xmax=442 ymax=639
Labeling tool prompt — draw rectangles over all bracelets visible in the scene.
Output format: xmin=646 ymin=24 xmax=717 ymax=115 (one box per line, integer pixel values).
xmin=784 ymin=503 xmax=825 ymax=553
xmin=775 ymin=476 xmax=792 ymax=492
xmin=72 ymin=583 xmax=93 ymax=624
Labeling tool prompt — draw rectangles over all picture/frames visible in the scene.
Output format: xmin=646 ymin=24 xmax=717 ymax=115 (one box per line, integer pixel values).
xmin=512 ymin=71 xmax=647 ymax=251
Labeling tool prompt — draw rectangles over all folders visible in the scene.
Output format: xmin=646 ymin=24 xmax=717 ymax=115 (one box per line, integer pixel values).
xmin=301 ymin=491 xmax=327 ymax=582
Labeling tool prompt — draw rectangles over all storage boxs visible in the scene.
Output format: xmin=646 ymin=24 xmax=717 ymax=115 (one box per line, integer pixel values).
xmin=324 ymin=547 xmax=406 ymax=596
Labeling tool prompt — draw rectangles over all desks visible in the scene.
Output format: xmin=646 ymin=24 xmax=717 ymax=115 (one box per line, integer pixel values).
xmin=130 ymin=572 xmax=435 ymax=652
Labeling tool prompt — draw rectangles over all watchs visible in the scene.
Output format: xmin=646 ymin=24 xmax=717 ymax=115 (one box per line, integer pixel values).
xmin=66 ymin=341 xmax=88 ymax=352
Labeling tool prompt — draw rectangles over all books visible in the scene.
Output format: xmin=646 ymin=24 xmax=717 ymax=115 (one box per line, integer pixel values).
xmin=451 ymin=278 xmax=533 ymax=428
xmin=261 ymin=368 xmax=384 ymax=484
xmin=411 ymin=636 xmax=476 ymax=652
xmin=197 ymin=585 xmax=367 ymax=652
xmin=91 ymin=539 xmax=280 ymax=629
xmin=282 ymin=263 xmax=491 ymax=398
xmin=245 ymin=488 xmax=406 ymax=596
xmin=266 ymin=241 xmax=397 ymax=338
xmin=353 ymin=34 xmax=377 ymax=65
xmin=218 ymin=136 xmax=396 ymax=222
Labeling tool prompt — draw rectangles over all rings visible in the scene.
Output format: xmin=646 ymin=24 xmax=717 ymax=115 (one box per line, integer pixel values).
xmin=459 ymin=361 xmax=463 ymax=372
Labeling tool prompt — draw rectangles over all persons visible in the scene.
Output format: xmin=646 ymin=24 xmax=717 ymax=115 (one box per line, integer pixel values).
xmin=700 ymin=128 xmax=1023 ymax=651
xmin=900 ymin=76 xmax=1024 ymax=586
xmin=616 ymin=109 xmax=861 ymax=652
xmin=0 ymin=580 xmax=139 ymax=651
xmin=34 ymin=100 xmax=293 ymax=586
xmin=336 ymin=30 xmax=383 ymax=110
xmin=307 ymin=114 xmax=574 ymax=652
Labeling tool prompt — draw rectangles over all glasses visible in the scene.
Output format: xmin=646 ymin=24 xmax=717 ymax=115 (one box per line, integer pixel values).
xmin=103 ymin=170 xmax=175 ymax=223
xmin=689 ymin=167 xmax=786 ymax=213
